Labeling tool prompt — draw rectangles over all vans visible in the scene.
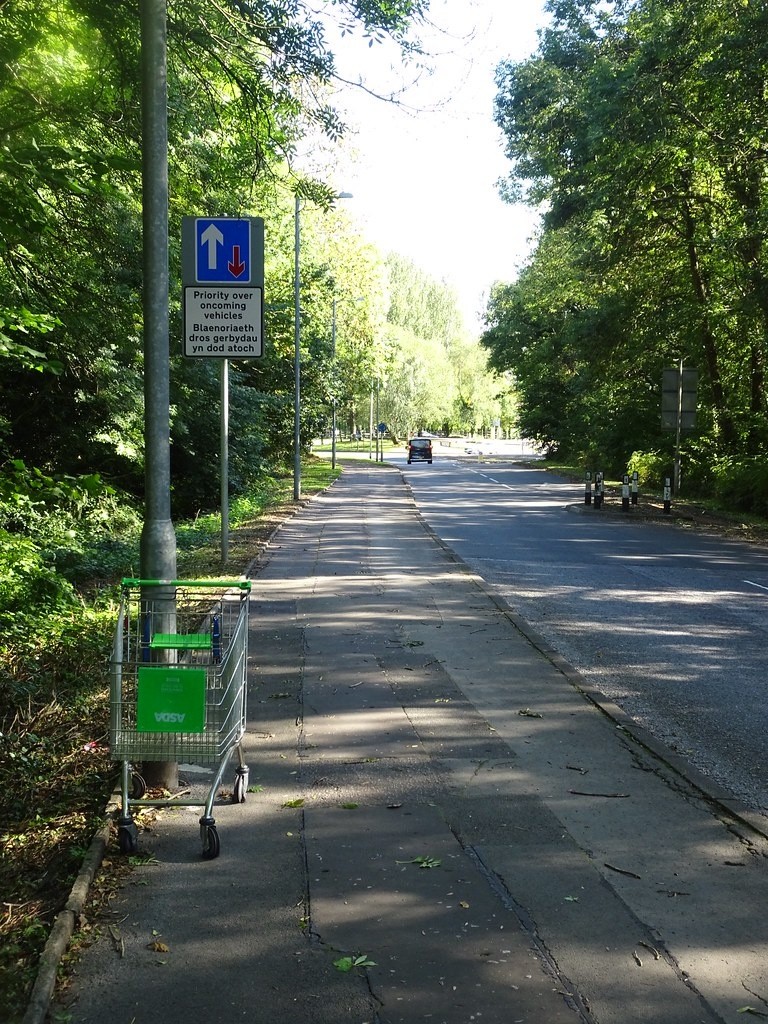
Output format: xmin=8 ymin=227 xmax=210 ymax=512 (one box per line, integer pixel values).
xmin=405 ymin=439 xmax=433 ymax=464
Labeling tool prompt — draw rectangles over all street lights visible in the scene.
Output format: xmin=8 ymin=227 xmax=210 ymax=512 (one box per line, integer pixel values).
xmin=330 ymin=296 xmax=367 ymax=470
xmin=290 ymin=189 xmax=355 ymax=500
xmin=369 ymin=362 xmax=388 ymax=459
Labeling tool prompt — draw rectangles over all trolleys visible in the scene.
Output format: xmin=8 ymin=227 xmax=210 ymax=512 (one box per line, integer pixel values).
xmin=107 ymin=577 xmax=252 ymax=860
